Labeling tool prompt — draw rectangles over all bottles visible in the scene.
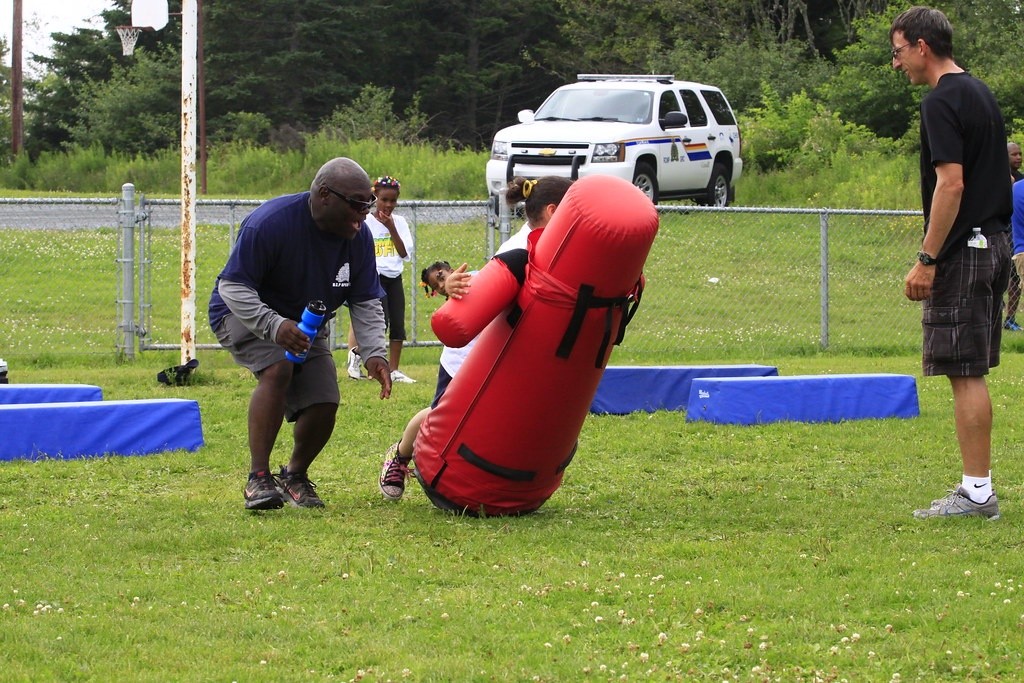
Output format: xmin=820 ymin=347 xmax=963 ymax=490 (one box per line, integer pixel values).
xmin=284 ymin=300 xmax=325 ymax=365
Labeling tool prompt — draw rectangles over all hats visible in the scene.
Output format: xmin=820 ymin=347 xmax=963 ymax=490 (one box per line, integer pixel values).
xmin=157 ymin=358 xmax=200 ymax=387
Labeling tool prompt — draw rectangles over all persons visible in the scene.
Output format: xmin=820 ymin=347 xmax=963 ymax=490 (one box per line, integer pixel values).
xmin=420 ymin=261 xmax=456 ymax=301
xmin=1002 ymin=142 xmax=1024 ymax=331
xmin=1012 ymin=177 xmax=1024 ymax=289
xmin=379 ymin=176 xmax=576 ymax=505
xmin=208 ymin=155 xmax=393 ymax=513
xmin=887 ymin=5 xmax=1013 ymax=521
xmin=347 ymin=176 xmax=418 ymax=384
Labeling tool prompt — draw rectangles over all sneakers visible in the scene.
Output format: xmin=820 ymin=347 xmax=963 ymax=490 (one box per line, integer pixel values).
xmin=347 ymin=347 xmax=367 ymax=380
xmin=389 ymin=370 xmax=418 ymax=385
xmin=242 ymin=471 xmax=284 ymax=510
xmin=380 ymin=438 xmax=413 ymax=501
xmin=274 ymin=464 xmax=325 ymax=511
xmin=910 ymin=485 xmax=1002 ymax=523
xmin=1003 ymin=320 xmax=1024 ymax=333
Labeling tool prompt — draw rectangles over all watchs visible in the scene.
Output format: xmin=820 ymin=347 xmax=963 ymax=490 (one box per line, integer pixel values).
xmin=916 ymin=251 xmax=938 ymax=266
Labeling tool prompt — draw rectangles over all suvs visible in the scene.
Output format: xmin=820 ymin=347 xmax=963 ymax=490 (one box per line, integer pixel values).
xmin=483 ymin=72 xmax=746 ymax=219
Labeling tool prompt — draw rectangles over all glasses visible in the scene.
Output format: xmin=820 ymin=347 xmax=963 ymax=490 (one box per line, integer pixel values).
xmin=325 ymin=185 xmax=377 ymax=210
xmin=891 ymin=42 xmax=916 ymax=58
